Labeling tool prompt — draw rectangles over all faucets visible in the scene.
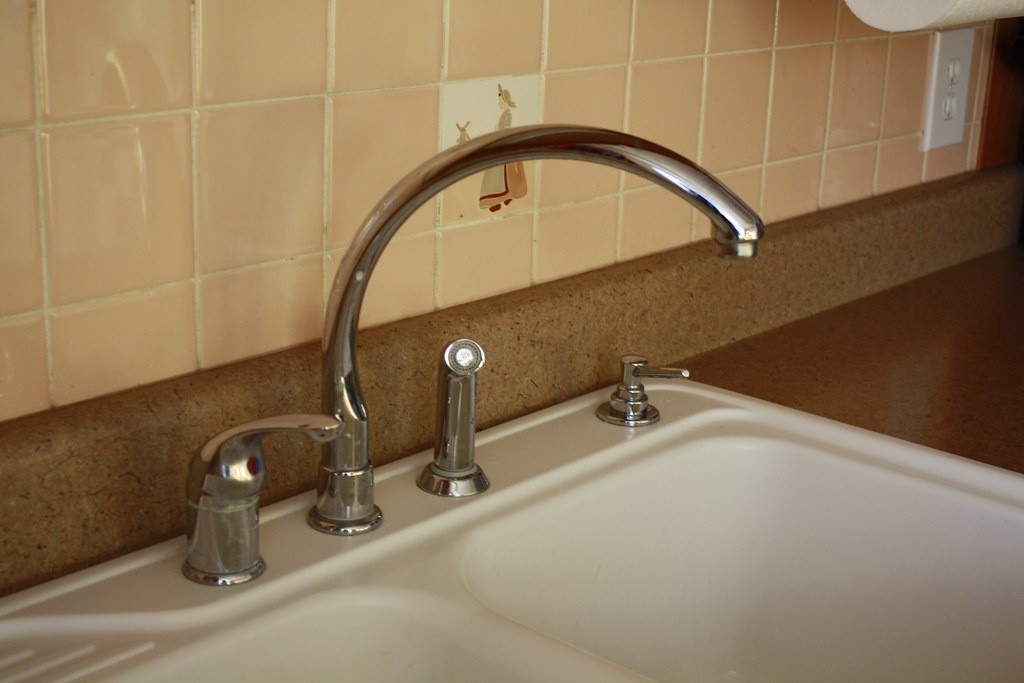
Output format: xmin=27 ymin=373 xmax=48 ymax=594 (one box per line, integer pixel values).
xmin=305 ymin=114 xmax=771 ymax=540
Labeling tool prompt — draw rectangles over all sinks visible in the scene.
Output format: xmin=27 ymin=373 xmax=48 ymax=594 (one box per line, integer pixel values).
xmin=91 ymin=589 xmax=566 ymax=680
xmin=451 ymin=406 xmax=1024 ymax=683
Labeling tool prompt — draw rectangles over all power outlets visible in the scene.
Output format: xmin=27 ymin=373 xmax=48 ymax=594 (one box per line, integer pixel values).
xmin=921 ymin=28 xmax=976 ymax=151
